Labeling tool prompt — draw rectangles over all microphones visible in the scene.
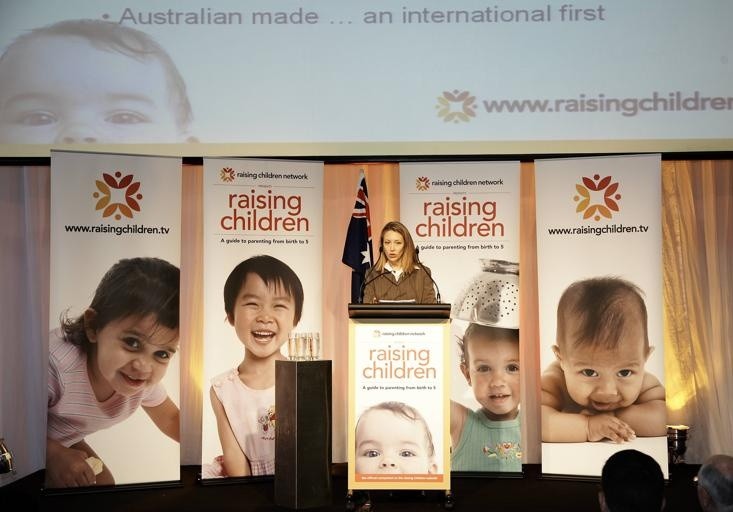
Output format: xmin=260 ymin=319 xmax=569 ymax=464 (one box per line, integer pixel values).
xmin=363 ymin=270 xmax=395 ymax=288
xmin=359 ymin=247 xmax=383 ymax=304
xmin=416 ymin=244 xmax=441 ymax=304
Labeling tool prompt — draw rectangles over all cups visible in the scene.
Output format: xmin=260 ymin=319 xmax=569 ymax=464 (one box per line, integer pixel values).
xmin=286 ymin=331 xmax=319 ymax=362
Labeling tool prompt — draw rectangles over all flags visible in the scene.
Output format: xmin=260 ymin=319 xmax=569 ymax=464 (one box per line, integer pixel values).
xmin=342 ymin=173 xmax=373 ymax=305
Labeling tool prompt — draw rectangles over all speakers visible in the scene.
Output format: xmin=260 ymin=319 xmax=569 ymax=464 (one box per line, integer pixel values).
xmin=274 ymin=360 xmax=332 ymax=512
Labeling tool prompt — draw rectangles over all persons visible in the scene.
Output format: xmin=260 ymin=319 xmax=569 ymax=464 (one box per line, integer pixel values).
xmin=0 ymin=18 xmax=197 ymax=146
xmin=355 ymin=402 xmax=438 ymax=475
xmin=362 ymin=221 xmax=437 ymax=304
xmin=697 ymin=454 xmax=733 ymax=512
xmin=45 ymin=257 xmax=181 ymax=488
xmin=210 ymin=255 xmax=304 ymax=478
xmin=540 ymin=275 xmax=666 ymax=445
xmin=598 ymin=449 xmax=667 ymax=512
xmin=449 ymin=322 xmax=523 ymax=473
